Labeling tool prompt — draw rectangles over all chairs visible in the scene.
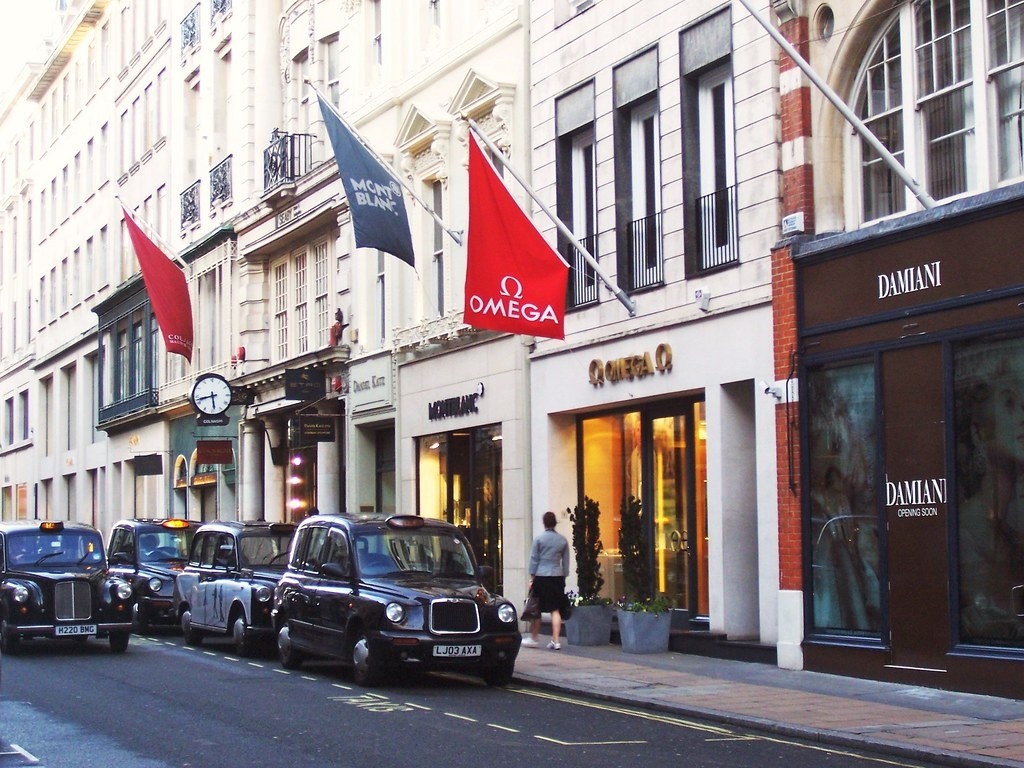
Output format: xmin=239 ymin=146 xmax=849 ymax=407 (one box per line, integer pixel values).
xmin=143 ymin=534 xmax=159 ymax=559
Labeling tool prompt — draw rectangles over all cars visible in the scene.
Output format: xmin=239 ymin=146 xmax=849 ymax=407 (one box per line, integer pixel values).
xmin=0 ymin=519 xmax=134 ymax=652
xmin=107 ymin=520 xmax=213 ymax=632
xmin=810 ymin=511 xmax=1008 ymax=639
xmin=174 ymin=519 xmax=297 ymax=656
xmin=271 ymin=511 xmax=521 ymax=688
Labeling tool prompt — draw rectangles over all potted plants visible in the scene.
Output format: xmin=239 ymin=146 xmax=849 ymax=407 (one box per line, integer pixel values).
xmin=614 ymin=495 xmax=673 ymax=655
xmin=562 ymin=496 xmax=614 ymax=646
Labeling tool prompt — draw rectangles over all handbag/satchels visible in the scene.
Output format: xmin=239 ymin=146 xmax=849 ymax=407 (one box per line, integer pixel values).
xmin=520 ymin=584 xmax=541 ymax=621
xmin=559 ymin=593 xmax=571 ymax=620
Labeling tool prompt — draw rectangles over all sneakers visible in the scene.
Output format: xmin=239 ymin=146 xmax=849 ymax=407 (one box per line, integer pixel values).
xmin=520 ymin=637 xmax=539 ymax=648
xmin=546 ymin=640 xmax=561 ymax=649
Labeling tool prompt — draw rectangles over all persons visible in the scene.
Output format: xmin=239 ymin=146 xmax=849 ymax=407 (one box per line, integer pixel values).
xmin=521 ymin=512 xmax=569 ymax=650
xmin=305 ymin=507 xmax=319 ymax=518
xmin=257 ymin=518 xmax=266 ymax=523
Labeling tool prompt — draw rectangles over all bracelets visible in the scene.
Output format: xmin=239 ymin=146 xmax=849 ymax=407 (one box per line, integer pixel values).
xmin=530 ymin=580 xmax=533 ymax=582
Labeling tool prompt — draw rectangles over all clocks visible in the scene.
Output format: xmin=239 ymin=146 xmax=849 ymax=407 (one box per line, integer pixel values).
xmin=189 ymin=373 xmax=232 ymax=416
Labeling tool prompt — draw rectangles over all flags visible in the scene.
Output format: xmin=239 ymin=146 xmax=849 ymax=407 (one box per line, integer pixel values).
xmin=122 ymin=205 xmax=193 ymax=364
xmin=464 ymin=130 xmax=568 ymax=340
xmin=317 ymin=94 xmax=415 ymax=268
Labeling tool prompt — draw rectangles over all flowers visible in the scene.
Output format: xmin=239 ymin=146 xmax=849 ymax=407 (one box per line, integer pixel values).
xmin=566 ymin=591 xmax=613 ymax=607
xmin=614 ymin=594 xmax=675 ymax=622
